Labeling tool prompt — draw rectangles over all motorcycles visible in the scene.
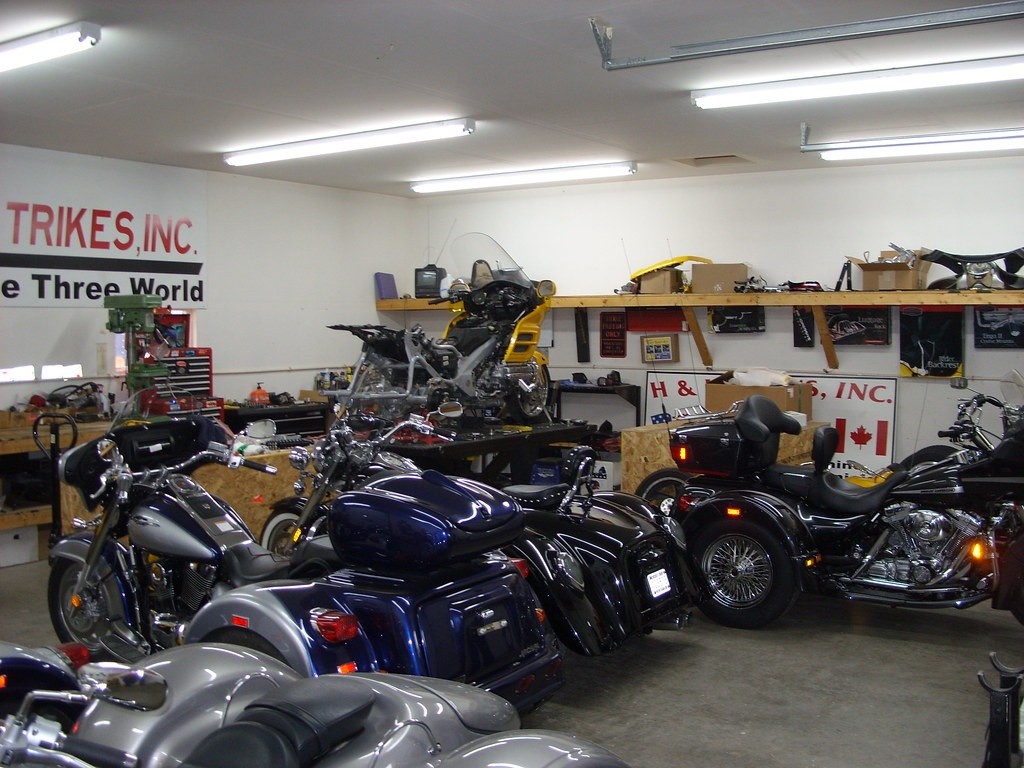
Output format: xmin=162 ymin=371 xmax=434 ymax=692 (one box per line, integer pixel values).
xmin=1 ymin=232 xmax=1024 ymax=768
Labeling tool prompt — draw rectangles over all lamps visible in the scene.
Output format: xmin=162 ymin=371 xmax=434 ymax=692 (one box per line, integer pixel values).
xmin=411 ymin=158 xmax=638 ymax=195
xmin=0 ymin=20 xmax=101 ymax=74
xmin=689 ymin=55 xmax=1024 ymax=110
xmin=222 ymin=116 xmax=475 ymax=168
xmin=817 ymin=136 xmax=1024 ymax=162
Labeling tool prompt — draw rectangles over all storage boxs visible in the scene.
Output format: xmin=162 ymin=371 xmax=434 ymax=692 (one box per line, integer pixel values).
xmin=844 ymin=250 xmax=933 ymax=290
xmin=691 ymin=263 xmax=748 ymax=292
xmin=372 ymin=289 xmax=1024 ymax=370
xmin=705 ymin=371 xmax=812 ymax=425
xmin=639 ymin=270 xmax=683 ymax=294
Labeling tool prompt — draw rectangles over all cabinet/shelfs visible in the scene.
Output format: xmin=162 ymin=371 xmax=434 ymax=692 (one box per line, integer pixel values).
xmin=0 ymin=389 xmax=382 ymax=533
